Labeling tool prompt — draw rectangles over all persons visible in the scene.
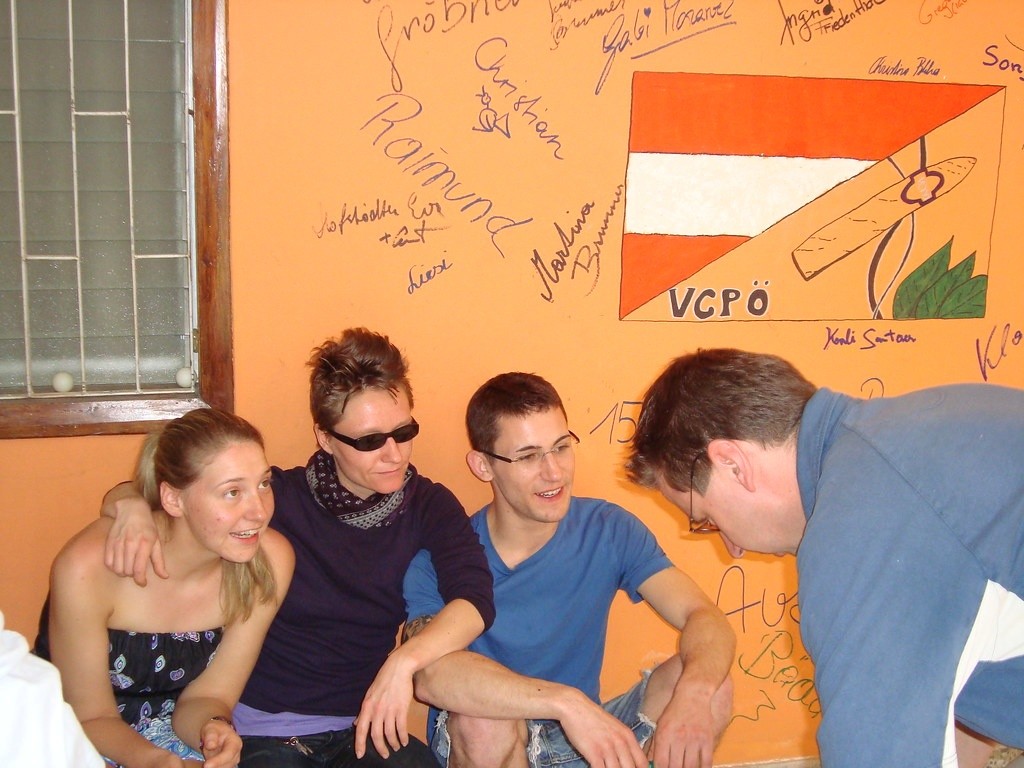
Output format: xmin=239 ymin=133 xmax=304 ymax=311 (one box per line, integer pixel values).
xmin=400 ymin=371 xmax=737 ymax=768
xmin=620 ymin=345 xmax=1024 ymax=768
xmin=100 ymin=325 xmax=497 ymax=768
xmin=30 ymin=408 xmax=296 ymax=768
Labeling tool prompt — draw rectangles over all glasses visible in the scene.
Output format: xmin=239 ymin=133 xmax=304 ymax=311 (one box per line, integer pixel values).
xmin=688 ymin=451 xmax=720 ymax=535
xmin=327 ymin=415 xmax=420 ymax=452
xmin=479 ymin=430 xmax=580 ymax=471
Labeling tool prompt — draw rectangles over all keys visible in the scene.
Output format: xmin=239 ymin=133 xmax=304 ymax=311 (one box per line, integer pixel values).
xmin=286 ymin=736 xmax=314 ymax=756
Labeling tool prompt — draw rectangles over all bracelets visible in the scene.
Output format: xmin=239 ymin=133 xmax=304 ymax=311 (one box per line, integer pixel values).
xmin=199 ymin=715 xmax=237 ymax=754
